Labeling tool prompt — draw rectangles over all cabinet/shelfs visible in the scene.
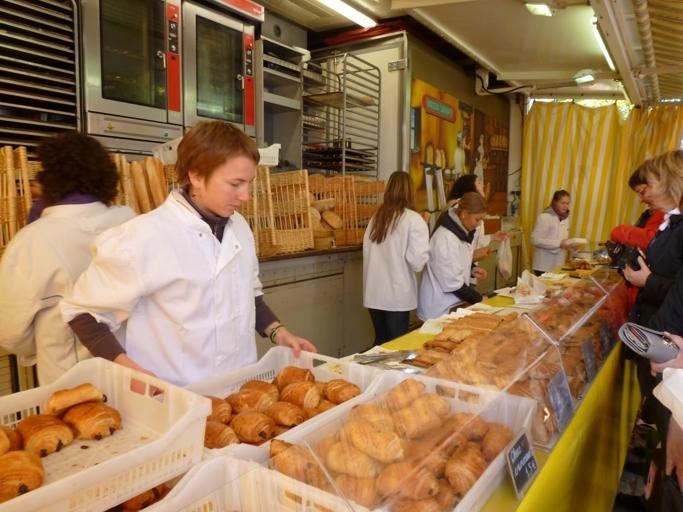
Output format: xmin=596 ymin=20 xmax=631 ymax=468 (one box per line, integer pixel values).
xmin=302 ymin=54 xmax=380 ymax=176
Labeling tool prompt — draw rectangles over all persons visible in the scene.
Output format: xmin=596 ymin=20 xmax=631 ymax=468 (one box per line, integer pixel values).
xmin=465 ymin=135 xmax=473 ymax=167
xmin=454 ymin=132 xmax=465 ymax=180
xmin=446 ymin=173 xmax=508 ymax=288
xmin=416 ymin=191 xmax=489 ymax=327
xmin=58 ymin=119 xmax=317 ymax=387
xmin=360 ymin=170 xmax=430 ymax=346
xmin=529 ymin=189 xmax=579 ymax=277
xmin=472 ymin=133 xmax=491 ymax=186
xmin=610 ymin=148 xmax=683 ymax=494
xmin=0 ymin=130 xmax=140 ymax=388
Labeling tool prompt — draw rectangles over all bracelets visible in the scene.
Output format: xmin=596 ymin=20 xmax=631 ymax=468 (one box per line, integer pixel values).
xmin=270 ymin=324 xmax=286 ymax=344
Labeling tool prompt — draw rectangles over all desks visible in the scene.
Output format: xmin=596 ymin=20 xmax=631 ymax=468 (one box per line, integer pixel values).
xmin=332 ymin=265 xmax=642 ymax=511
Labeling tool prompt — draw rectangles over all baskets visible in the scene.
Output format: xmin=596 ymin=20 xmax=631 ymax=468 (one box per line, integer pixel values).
xmin=0 ymin=345 xmax=539 ymax=512
xmin=0 ymin=345 xmax=539 ymax=511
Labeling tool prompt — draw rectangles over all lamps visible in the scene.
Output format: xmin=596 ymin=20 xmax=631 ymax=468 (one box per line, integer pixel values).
xmin=574 ymin=71 xmax=594 ymax=83
xmin=525 ymin=4 xmax=552 ymax=17
xmin=591 ymin=19 xmax=615 ymax=70
xmin=316 ymin=1 xmax=376 ymax=29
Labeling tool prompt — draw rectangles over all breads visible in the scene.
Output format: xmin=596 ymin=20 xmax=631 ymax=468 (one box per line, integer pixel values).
xmin=272 ymin=190 xmax=343 ymax=229
xmin=0 ymin=383 xmax=122 ymax=503
xmin=125 ymin=158 xmax=168 ymax=215
xmin=104 ymin=484 xmax=171 ymax=512
xmin=270 ymin=377 xmax=515 ymax=512
xmin=202 ymin=366 xmax=360 ymax=450
xmin=401 ymin=258 xmax=629 ymax=445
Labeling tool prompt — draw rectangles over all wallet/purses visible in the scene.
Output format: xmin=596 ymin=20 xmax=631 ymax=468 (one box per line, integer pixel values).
xmin=619 ymin=323 xmax=678 ymax=363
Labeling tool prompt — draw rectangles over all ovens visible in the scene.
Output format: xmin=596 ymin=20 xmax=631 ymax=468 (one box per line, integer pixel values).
xmin=76 ymin=0 xmax=263 ymax=163
